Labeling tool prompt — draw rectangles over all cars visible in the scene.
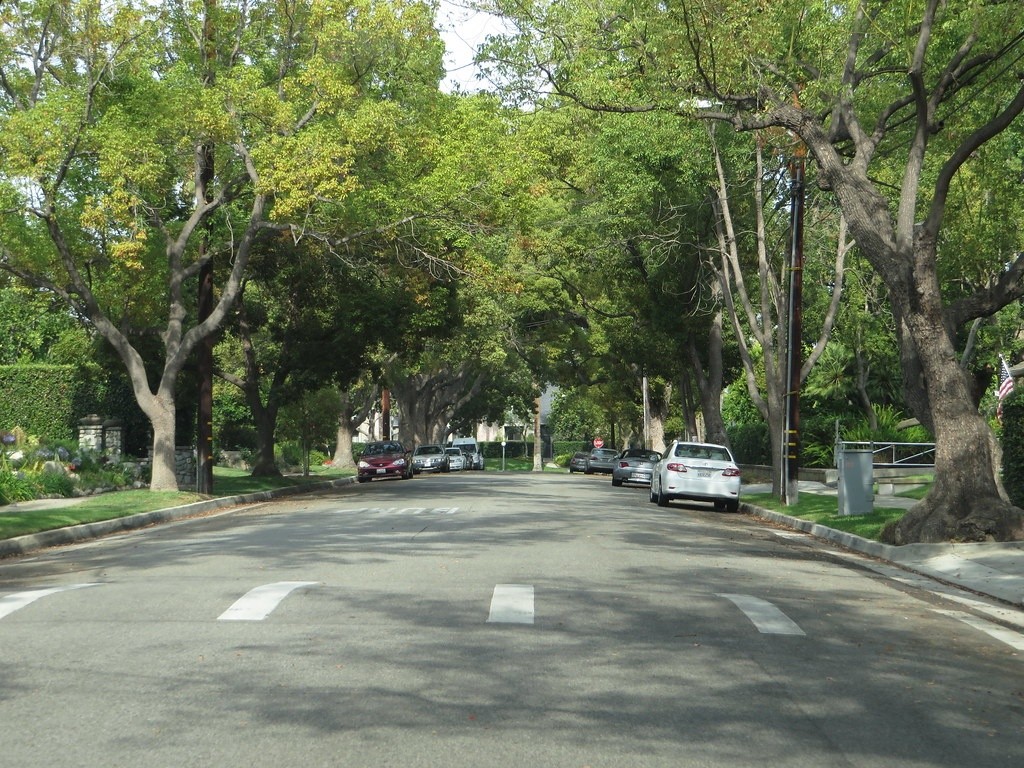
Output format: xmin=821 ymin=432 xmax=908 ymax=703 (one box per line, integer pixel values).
xmin=570 ymin=448 xmax=619 ymax=473
xmin=649 ymin=439 xmax=741 ymax=512
xmin=412 ymin=437 xmax=484 ymax=474
xmin=612 ymin=449 xmax=663 ymax=486
xmin=356 ymin=440 xmax=412 ymax=483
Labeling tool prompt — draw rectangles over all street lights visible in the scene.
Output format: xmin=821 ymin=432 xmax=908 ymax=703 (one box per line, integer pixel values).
xmin=678 ymin=84 xmax=807 ymax=506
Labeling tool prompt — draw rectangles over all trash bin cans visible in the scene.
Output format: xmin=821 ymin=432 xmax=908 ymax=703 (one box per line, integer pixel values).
xmin=836 ymin=438 xmax=875 ymax=516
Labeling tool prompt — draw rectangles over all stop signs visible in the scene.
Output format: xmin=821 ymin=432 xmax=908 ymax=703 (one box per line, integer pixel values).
xmin=593 ymin=437 xmax=604 ymax=448
